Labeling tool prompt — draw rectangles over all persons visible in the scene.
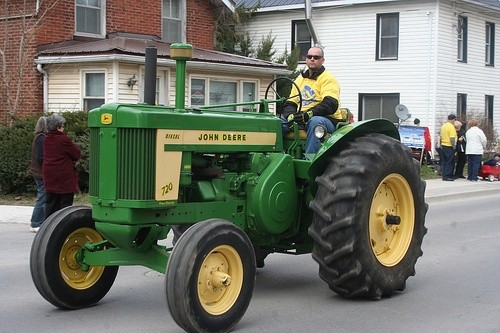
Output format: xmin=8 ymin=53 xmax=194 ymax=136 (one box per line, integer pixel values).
xmin=278 ymin=47 xmax=344 ymax=160
xmin=465 ymin=119 xmax=487 ymax=181
xmin=29 ymin=117 xmax=50 ymax=232
xmin=43 ymin=114 xmax=81 ymax=221
xmin=436 ymin=114 xmax=466 ymax=181
xmin=483 ymin=153 xmax=500 ymax=167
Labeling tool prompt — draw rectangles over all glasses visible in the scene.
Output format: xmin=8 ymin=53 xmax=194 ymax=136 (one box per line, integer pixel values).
xmin=307 ymin=55 xmax=323 ymax=60
xmin=57 ymin=125 xmax=65 ymax=128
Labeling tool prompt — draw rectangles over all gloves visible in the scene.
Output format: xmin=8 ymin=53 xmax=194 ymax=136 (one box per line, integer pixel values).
xmin=287 ymin=111 xmax=310 ymax=128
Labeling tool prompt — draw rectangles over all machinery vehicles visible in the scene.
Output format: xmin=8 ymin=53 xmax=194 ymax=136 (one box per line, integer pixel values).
xmin=27 ymin=44 xmax=428 ymax=333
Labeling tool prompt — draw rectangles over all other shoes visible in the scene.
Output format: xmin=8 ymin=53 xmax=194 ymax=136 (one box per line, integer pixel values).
xmin=460 ymin=176 xmax=466 ymax=178
xmin=444 ymin=178 xmax=454 ymax=181
xmin=452 ymin=176 xmax=458 ymax=179
xmin=30 ymin=226 xmax=41 ymax=232
